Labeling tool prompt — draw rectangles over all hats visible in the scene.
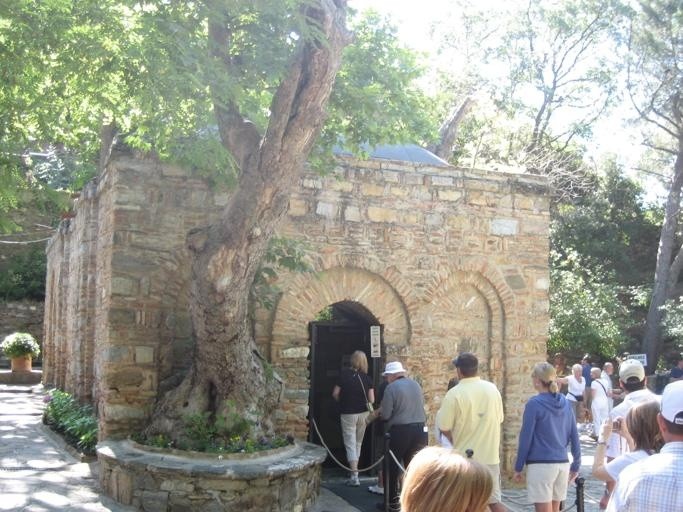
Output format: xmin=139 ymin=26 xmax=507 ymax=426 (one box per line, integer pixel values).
xmin=660 ymin=380 xmax=683 ymax=425
xmin=451 ymin=353 xmax=478 ymax=370
xmin=382 ymin=361 xmax=406 ymax=376
xmin=620 ymin=360 xmax=645 ymax=384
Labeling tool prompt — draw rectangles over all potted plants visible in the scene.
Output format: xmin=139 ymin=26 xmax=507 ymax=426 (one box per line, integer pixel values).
xmin=1 ymin=333 xmax=40 ymax=372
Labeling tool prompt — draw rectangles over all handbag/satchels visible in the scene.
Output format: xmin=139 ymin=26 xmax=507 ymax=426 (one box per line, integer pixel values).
xmin=574 ymin=396 xmax=583 ymax=400
xmin=367 ymin=403 xmax=374 ymax=412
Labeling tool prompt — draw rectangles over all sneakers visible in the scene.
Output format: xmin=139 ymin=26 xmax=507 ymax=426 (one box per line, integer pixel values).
xmin=368 ymin=485 xmax=384 ymax=495
xmin=345 ymin=478 xmax=360 ymax=487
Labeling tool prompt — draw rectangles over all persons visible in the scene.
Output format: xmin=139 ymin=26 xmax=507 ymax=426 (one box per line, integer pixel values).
xmin=373 ymin=351 xmax=513 ymax=512
xmin=332 ymin=350 xmax=376 ymax=488
xmin=512 ymin=361 xmax=582 ymax=512
xmin=551 ymin=351 xmax=683 ymax=512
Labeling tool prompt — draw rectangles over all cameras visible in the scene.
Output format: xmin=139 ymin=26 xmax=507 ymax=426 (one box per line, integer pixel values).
xmin=603 ymin=419 xmax=620 ymax=431
